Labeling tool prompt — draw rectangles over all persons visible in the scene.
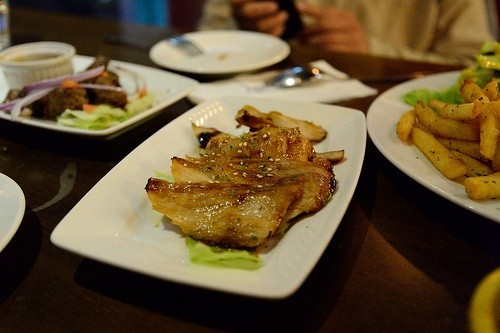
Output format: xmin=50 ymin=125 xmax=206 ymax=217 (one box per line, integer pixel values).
xmin=198 ymin=0 xmax=500 ymax=64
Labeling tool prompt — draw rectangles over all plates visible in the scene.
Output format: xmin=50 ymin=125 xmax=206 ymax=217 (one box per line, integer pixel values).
xmin=185 ymin=60 xmax=378 ymax=102
xmin=0 ymin=54 xmax=200 ymax=141
xmin=148 ymin=30 xmax=292 ymax=74
xmin=51 ymin=93 xmax=367 ymax=299
xmin=366 ymin=67 xmax=500 ymax=223
xmin=469 ymin=268 xmax=500 ymax=333
xmin=0 ymin=173 xmax=27 ymax=252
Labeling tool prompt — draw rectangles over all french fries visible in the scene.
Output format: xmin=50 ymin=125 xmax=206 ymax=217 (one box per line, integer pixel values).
xmin=396 ymin=79 xmax=500 ymax=200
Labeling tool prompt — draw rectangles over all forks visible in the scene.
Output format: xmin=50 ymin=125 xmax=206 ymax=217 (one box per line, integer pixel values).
xmin=98 ymin=33 xmax=202 ymax=57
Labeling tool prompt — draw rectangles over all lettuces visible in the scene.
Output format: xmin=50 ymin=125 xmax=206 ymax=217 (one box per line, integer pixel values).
xmin=185 ymin=235 xmax=260 ymax=269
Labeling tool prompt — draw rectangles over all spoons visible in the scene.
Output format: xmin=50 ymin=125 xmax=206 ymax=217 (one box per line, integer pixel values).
xmin=262 ymin=66 xmax=426 ymax=90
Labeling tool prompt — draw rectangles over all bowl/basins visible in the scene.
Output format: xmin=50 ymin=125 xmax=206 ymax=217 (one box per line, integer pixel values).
xmin=0 ymin=40 xmax=76 ymax=87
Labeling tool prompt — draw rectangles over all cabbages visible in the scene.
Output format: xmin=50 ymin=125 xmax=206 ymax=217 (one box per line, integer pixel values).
xmin=399 ymin=38 xmax=500 ymax=109
xmin=57 ymin=89 xmax=155 ymax=129
